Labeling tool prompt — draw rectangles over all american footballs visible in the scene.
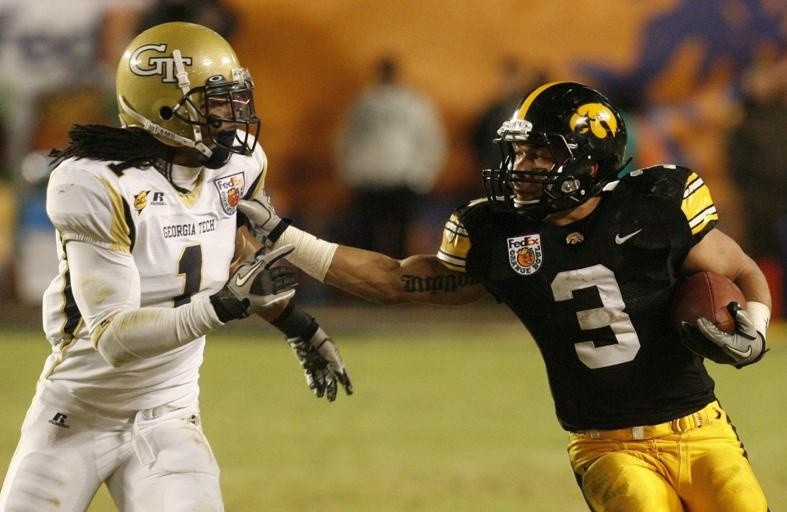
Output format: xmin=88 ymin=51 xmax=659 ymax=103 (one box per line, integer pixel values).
xmin=668 ymin=272 xmax=747 ymax=336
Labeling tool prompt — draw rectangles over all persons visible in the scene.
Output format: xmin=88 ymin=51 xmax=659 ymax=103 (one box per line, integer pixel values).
xmin=727 ymin=60 xmax=785 ymax=322
xmin=0 ymin=19 xmax=357 ymax=510
xmin=221 ymin=77 xmax=775 ymax=512
xmin=328 ymin=51 xmax=452 ymax=271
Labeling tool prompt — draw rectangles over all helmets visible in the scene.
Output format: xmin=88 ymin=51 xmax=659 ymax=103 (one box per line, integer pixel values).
xmin=116 ymin=20 xmax=259 ymax=161
xmin=483 ymin=81 xmax=627 ymax=203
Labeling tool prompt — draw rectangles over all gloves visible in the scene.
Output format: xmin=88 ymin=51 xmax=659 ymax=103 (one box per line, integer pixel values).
xmin=678 ymin=299 xmax=772 ymax=368
xmin=212 ymin=242 xmax=296 ymax=320
xmin=285 ymin=322 xmax=353 ymax=402
